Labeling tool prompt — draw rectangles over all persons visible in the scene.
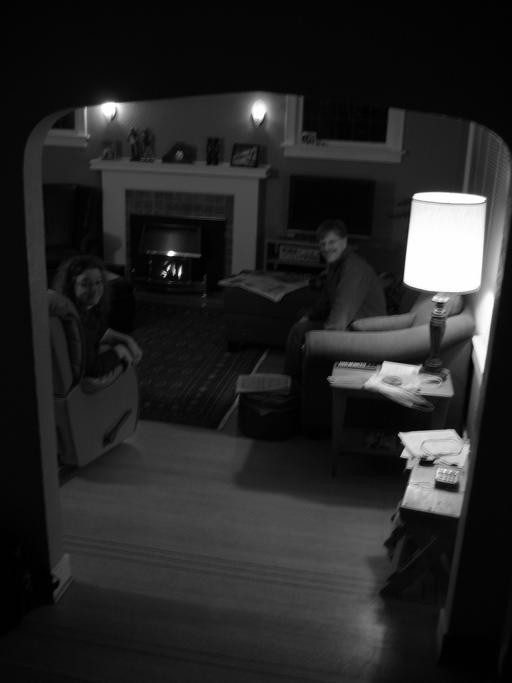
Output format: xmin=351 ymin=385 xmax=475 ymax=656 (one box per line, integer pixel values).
xmin=54 ymin=254 xmax=137 ymax=378
xmin=282 ymin=220 xmax=386 ymax=395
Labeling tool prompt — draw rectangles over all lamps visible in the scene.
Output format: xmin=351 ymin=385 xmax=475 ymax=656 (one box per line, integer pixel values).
xmin=402 ymin=192 xmax=488 ymax=378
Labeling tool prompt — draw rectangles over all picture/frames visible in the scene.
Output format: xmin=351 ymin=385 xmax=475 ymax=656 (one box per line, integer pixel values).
xmin=231 ymin=143 xmax=260 ymax=168
xmin=42 ymin=107 xmax=91 ymax=150
xmin=282 ymin=94 xmax=406 ymax=165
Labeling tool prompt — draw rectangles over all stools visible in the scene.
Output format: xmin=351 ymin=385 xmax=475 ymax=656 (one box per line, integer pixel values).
xmin=238 ymin=390 xmax=296 ymax=441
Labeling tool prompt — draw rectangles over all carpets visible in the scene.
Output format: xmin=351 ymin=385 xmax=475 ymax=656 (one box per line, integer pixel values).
xmin=119 ymin=308 xmax=270 ymax=431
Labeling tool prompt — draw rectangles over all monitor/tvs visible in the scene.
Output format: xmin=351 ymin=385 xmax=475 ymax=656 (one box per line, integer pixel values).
xmin=285 ymin=173 xmax=377 ymax=241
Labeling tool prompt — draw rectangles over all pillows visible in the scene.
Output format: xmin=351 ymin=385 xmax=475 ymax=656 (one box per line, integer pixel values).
xmin=348 ymin=313 xmax=416 ymax=331
xmin=409 ymin=293 xmax=462 ymax=326
xmin=48 ymin=290 xmax=87 ymax=381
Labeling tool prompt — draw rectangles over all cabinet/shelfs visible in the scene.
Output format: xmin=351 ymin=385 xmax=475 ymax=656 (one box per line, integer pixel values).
xmin=263 ymin=239 xmax=328 ymax=274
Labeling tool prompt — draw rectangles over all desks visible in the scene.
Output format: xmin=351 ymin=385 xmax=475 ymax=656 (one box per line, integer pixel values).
xmin=379 ymin=460 xmax=466 ymax=604
xmin=331 ymin=363 xmax=455 ymax=480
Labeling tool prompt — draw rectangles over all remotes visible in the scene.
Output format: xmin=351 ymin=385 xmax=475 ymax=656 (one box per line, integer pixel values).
xmin=336 ymin=359 xmax=379 ymax=369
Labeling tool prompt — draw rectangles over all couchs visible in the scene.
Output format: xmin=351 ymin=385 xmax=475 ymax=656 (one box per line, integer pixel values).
xmin=48 ymin=288 xmax=142 ymax=468
xmin=299 ymin=294 xmax=476 ymax=440
